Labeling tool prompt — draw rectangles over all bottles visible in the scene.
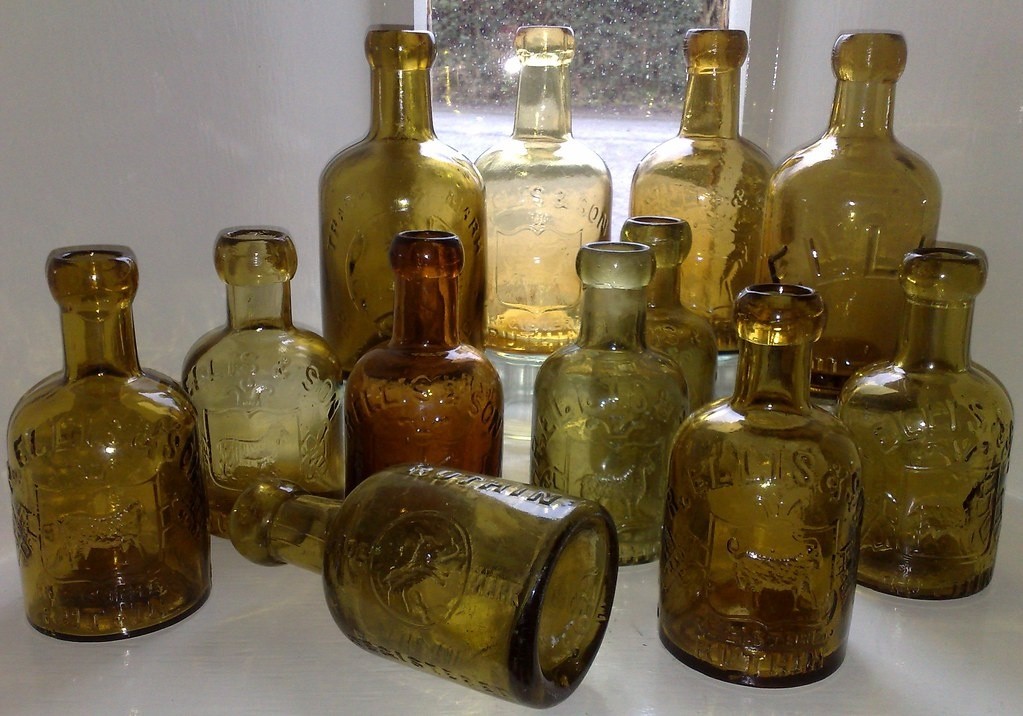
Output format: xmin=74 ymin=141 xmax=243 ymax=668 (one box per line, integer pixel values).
xmin=628 ymin=28 xmax=779 ymax=353
xmin=346 ymin=232 xmax=504 ymax=487
xmin=8 ymin=246 xmax=213 ymax=644
xmin=657 ymin=281 xmax=862 ymax=691
xmin=766 ymin=31 xmax=943 ymax=399
xmin=316 ymin=28 xmax=487 ymax=377
xmin=620 ymin=215 xmax=715 ymax=420
xmin=837 ymin=242 xmax=1014 ymax=600
xmin=228 ymin=462 xmax=620 ymax=710
xmin=183 ymin=226 xmax=348 ymax=539
xmin=468 ymin=25 xmax=614 ymax=357
xmin=531 ymin=241 xmax=687 ymax=567
